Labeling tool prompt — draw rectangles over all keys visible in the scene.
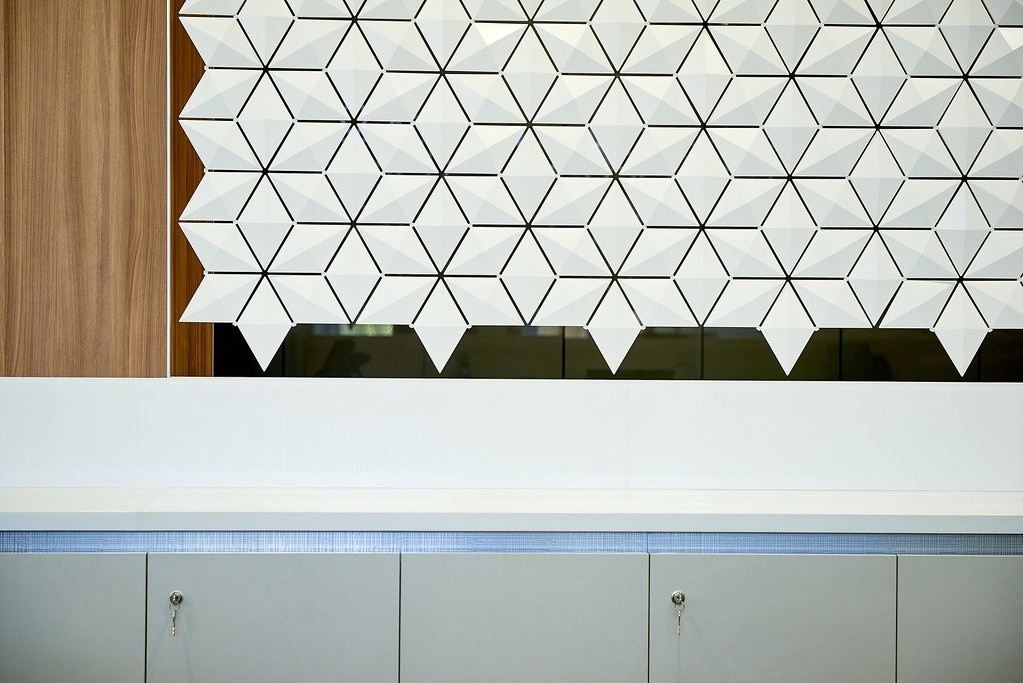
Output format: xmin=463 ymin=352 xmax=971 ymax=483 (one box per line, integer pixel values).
xmin=678 ymin=610 xmax=681 ymax=636
xmin=172 ymin=609 xmax=176 ymax=637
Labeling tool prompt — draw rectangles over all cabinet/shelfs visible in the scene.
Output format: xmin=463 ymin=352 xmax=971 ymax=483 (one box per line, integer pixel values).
xmin=1 ymin=489 xmax=1023 ymax=683
